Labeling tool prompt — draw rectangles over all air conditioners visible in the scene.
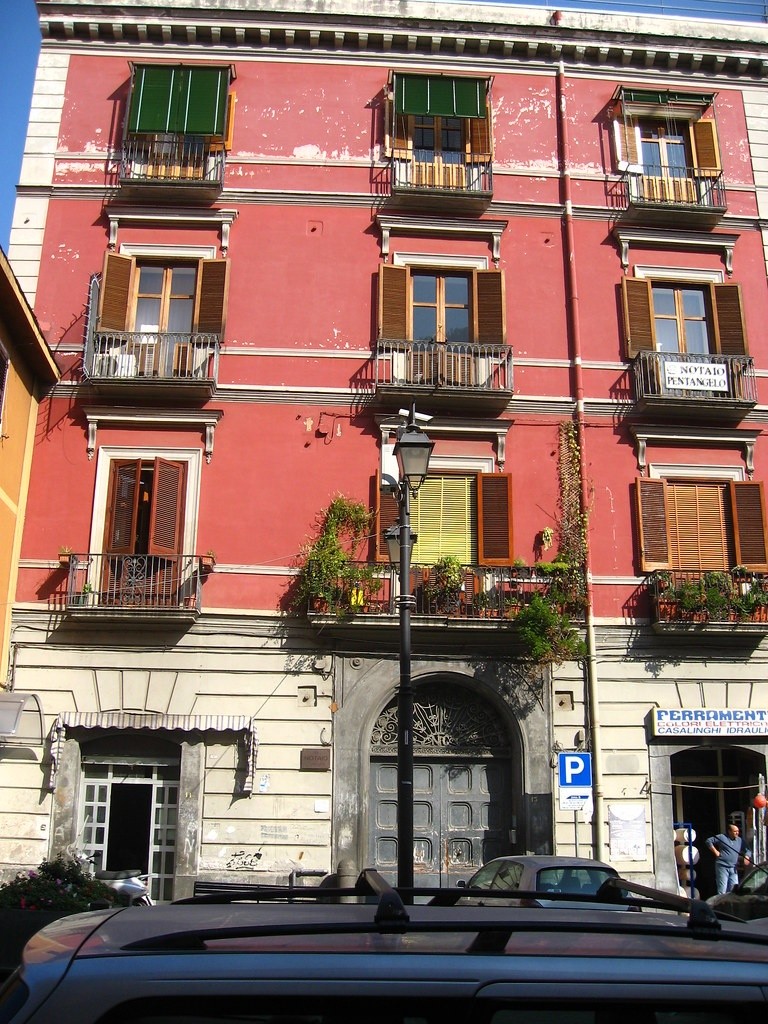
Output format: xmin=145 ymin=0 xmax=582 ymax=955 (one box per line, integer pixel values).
xmin=92 ymin=354 xmax=137 ymax=378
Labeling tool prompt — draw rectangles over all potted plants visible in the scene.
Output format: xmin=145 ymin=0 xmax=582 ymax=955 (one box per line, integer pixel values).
xmin=274 ymin=491 xmax=390 ymax=639
xmin=79 ymin=583 xmax=92 ymax=604
xmin=58 ymin=546 xmax=76 ymax=568
xmin=653 ymin=565 xmax=768 ymax=623
xmin=472 ymin=559 xmax=588 ymax=666
xmin=414 ymin=555 xmax=466 ymax=615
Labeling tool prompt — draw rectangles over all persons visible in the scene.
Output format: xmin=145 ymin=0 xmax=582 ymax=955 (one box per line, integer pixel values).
xmin=706 ymin=824 xmax=752 ymax=896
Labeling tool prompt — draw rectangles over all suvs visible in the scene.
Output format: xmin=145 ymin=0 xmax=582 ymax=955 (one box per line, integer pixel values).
xmin=1 ymin=869 xmax=768 ymax=1024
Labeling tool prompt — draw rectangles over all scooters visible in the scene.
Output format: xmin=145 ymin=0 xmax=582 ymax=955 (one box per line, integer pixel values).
xmin=81 ymin=870 xmax=152 ymax=907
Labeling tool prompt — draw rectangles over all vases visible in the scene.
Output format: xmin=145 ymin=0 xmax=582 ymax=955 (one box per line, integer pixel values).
xmin=185 ymin=597 xmax=196 ymax=609
xmin=201 ymin=550 xmax=217 ymax=573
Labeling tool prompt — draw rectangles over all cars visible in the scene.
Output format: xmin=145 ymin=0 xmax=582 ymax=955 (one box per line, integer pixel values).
xmin=456 ymin=854 xmax=642 ymax=912
xmin=705 ymin=862 xmax=768 ymax=922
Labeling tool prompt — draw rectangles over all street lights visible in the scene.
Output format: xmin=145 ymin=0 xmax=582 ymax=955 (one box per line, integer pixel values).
xmin=392 ymin=402 xmax=433 ymax=905
